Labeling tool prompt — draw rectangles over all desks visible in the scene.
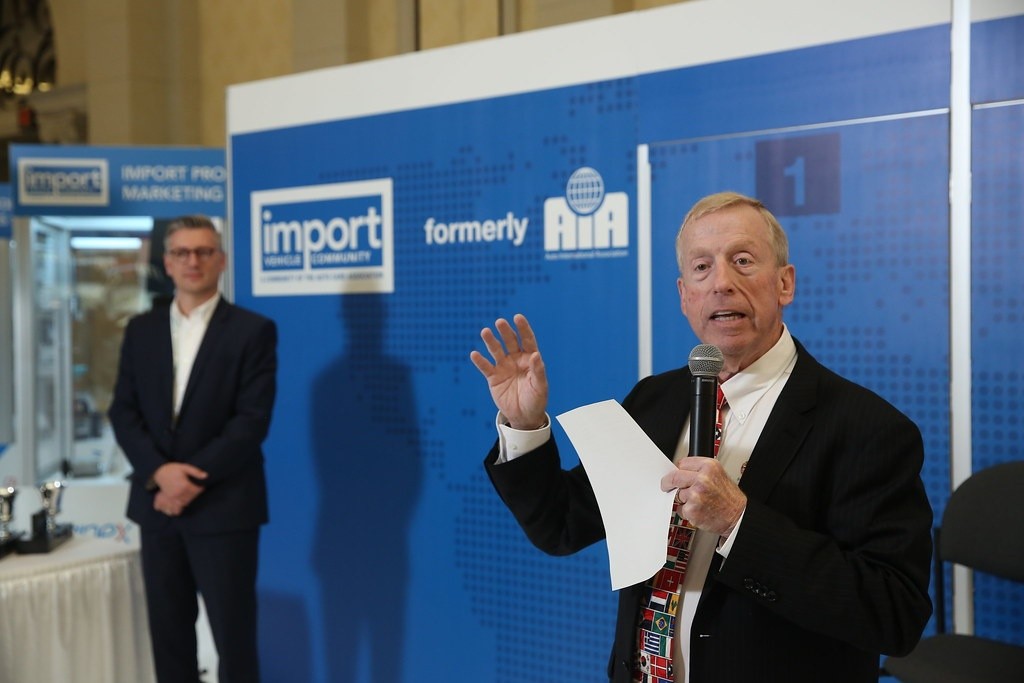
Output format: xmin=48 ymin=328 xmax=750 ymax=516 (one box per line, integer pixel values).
xmin=0 ymin=535 xmax=159 ymax=683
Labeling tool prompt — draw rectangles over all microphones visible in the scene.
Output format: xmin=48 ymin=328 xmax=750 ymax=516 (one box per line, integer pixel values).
xmin=687 ymin=345 xmax=725 ymax=459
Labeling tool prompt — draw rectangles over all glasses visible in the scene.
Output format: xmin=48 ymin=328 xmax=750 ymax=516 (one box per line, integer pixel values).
xmin=165 ymin=246 xmax=216 ymax=261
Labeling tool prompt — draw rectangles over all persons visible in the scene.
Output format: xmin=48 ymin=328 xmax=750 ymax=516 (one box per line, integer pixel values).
xmin=106 ymin=215 xmax=279 ymax=682
xmin=470 ymin=191 xmax=935 ymax=683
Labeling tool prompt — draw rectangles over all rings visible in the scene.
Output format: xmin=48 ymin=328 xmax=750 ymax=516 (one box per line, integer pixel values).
xmin=675 ymin=489 xmax=683 ymax=504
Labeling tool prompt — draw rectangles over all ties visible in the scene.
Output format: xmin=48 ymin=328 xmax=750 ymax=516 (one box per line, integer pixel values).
xmin=638 ymin=385 xmax=729 ymax=683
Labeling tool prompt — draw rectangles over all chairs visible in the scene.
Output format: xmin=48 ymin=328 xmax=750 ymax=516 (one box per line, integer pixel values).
xmin=879 ymin=460 xmax=1023 ymax=683
